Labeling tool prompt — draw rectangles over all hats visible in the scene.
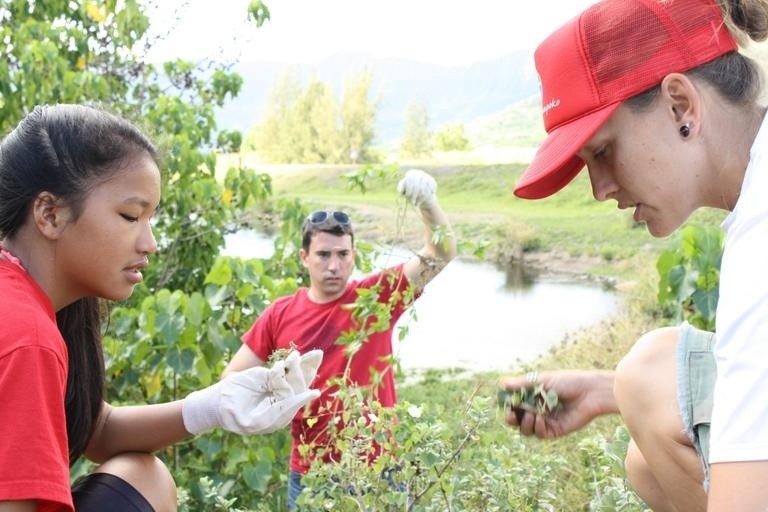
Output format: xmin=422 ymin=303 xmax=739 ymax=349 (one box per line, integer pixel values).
xmin=513 ymin=1 xmax=738 ymax=201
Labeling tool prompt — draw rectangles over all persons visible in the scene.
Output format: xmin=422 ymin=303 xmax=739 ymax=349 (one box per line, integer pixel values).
xmin=0 ymin=105 xmax=323 ymax=509
xmin=220 ymin=164 xmax=456 ymax=511
xmin=496 ymin=0 xmax=766 ymax=509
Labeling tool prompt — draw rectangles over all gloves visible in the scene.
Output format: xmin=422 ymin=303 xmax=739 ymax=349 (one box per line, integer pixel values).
xmin=181 ymin=348 xmax=325 ymax=438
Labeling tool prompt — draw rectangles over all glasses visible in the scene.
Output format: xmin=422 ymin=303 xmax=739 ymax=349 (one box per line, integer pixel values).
xmin=301 ymin=210 xmax=351 ymax=232
